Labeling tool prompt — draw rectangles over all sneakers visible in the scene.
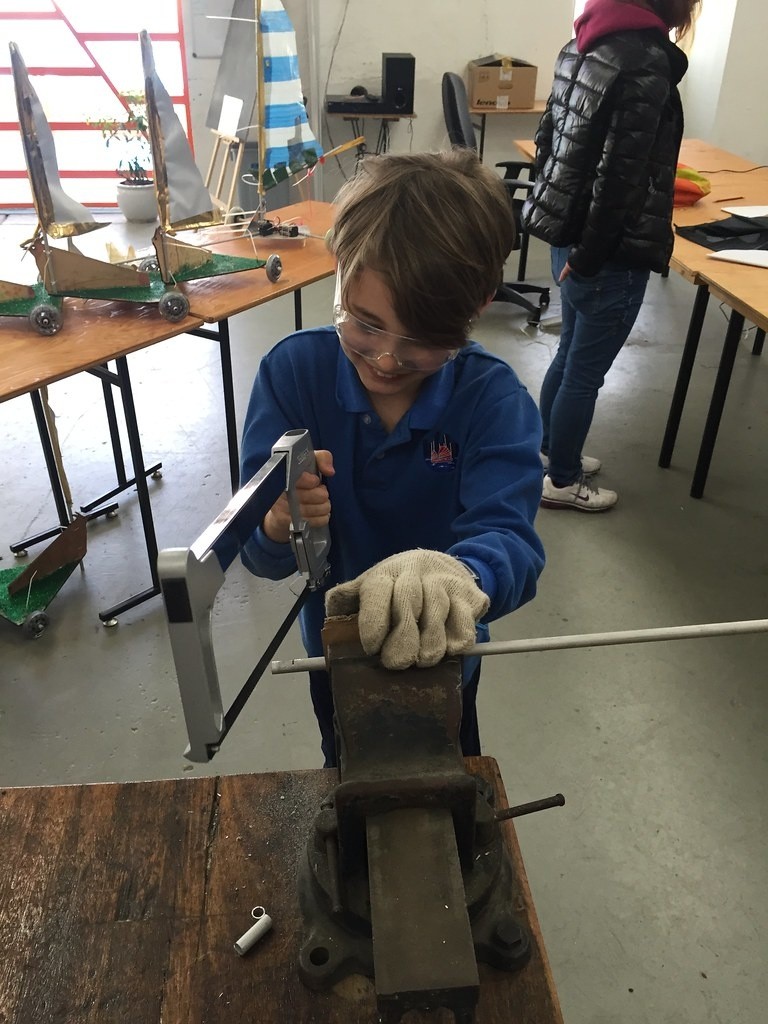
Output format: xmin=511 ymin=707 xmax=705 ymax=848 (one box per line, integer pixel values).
xmin=538 ymin=447 xmax=602 ymax=476
xmin=540 ymin=471 xmax=618 ymax=513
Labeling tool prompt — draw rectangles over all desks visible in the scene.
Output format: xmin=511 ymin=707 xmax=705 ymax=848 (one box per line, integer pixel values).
xmin=512 ymin=139 xmax=768 ymax=500
xmin=0 ymin=201 xmax=339 ymax=626
xmin=0 ymin=756 xmax=566 ymax=1024
xmin=326 ymin=113 xmax=417 ymax=178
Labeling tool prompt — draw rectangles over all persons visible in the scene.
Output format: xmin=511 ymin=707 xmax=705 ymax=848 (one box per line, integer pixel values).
xmin=237 ymin=153 xmax=546 ymax=773
xmin=523 ymin=0 xmax=703 ymax=513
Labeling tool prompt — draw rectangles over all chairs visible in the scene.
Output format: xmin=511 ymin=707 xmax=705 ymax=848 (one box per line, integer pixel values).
xmin=442 ymin=72 xmax=552 ymax=327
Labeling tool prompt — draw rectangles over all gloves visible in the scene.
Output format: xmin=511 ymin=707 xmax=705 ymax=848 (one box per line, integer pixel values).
xmin=324 ymin=550 xmax=490 ymax=671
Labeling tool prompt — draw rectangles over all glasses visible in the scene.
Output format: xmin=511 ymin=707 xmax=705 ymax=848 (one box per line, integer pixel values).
xmin=332 ymin=257 xmax=472 ymax=374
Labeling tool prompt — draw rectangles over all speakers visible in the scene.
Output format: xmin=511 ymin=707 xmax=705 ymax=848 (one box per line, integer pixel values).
xmin=382 ymin=53 xmax=416 ymax=114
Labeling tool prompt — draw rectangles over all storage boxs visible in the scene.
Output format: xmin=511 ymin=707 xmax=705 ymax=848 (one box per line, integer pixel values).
xmin=468 ymin=55 xmax=538 ymax=109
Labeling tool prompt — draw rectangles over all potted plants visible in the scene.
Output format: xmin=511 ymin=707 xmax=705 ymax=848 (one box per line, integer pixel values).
xmin=85 ymin=89 xmax=159 ymax=225
